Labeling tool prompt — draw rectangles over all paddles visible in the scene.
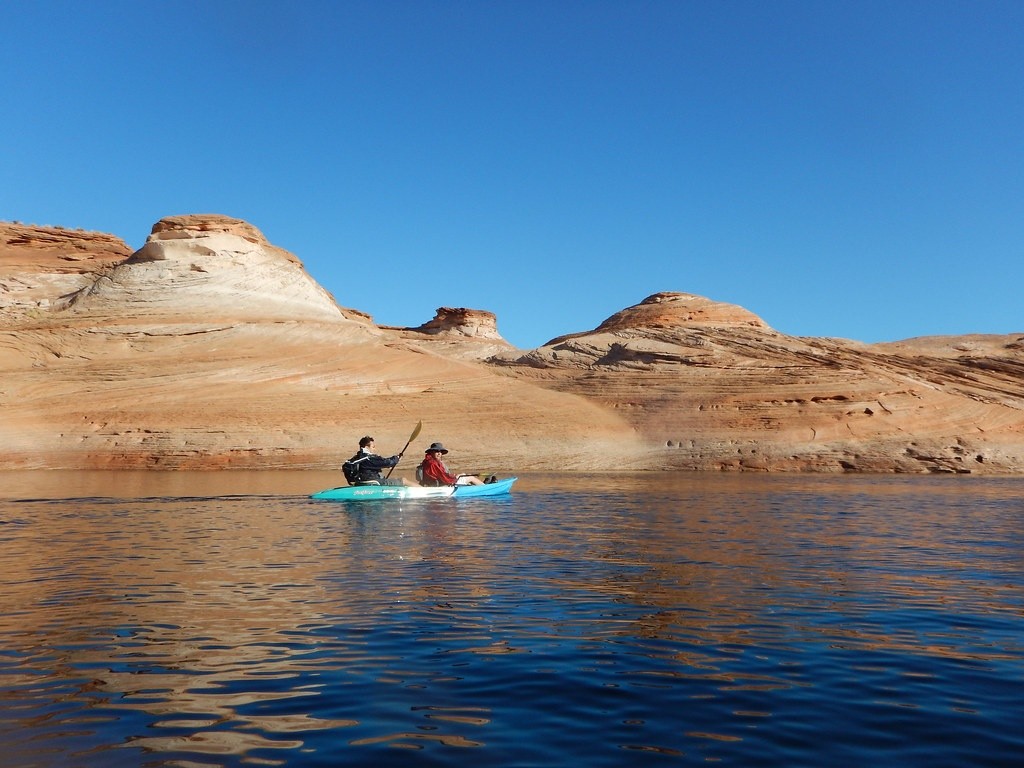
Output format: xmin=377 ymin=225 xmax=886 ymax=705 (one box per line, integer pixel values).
xmin=385 ymin=419 xmax=424 ymax=481
xmin=458 ymin=470 xmax=498 ymax=478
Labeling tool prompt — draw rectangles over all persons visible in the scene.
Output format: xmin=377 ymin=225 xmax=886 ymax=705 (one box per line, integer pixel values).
xmin=342 ymin=436 xmax=423 ymax=487
xmin=415 ymin=442 xmax=487 ymax=487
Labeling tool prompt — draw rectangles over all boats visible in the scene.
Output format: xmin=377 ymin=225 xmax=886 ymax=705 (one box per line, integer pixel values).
xmin=308 ymin=477 xmax=518 ymax=501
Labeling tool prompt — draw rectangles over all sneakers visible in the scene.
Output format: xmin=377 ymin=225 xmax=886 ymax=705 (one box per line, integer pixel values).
xmin=491 ymin=476 xmax=497 ymax=483
xmin=483 ymin=477 xmax=490 ymax=484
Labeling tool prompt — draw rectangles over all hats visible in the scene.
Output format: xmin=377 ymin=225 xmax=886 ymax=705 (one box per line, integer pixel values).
xmin=425 ymin=442 xmax=448 ymax=455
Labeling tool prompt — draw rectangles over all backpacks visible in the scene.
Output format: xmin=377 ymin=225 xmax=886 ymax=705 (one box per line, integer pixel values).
xmin=341 ymin=454 xmax=371 ymax=482
xmin=416 ymin=465 xmax=424 ymax=482
